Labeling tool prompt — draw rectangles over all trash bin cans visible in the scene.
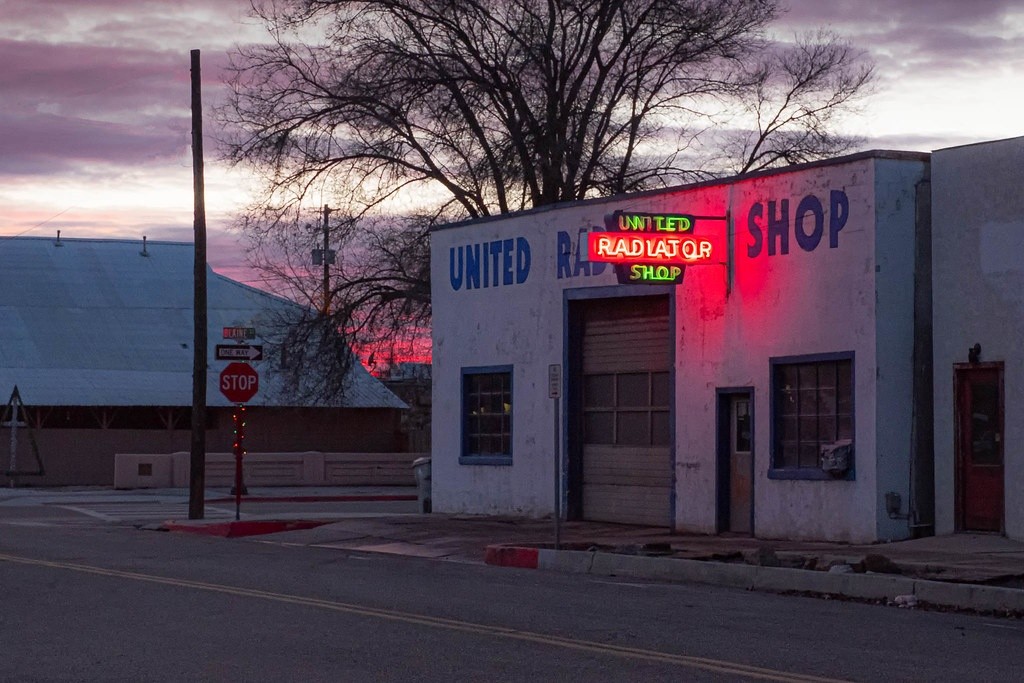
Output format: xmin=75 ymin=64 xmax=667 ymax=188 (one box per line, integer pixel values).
xmin=411 ymin=457 xmax=432 ymax=513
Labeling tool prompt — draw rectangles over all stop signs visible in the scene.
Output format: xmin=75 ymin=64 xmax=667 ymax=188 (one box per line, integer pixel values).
xmin=219 ymin=363 xmax=259 ymax=403
